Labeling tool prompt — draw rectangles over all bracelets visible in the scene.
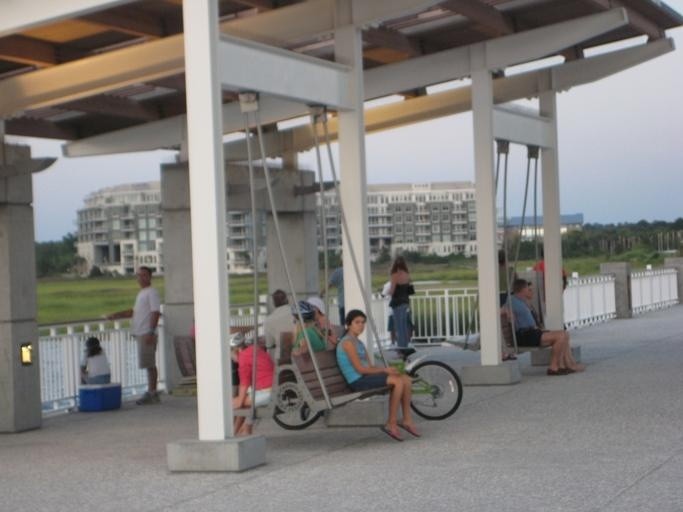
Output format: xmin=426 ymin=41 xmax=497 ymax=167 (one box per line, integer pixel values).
xmin=144 ymin=327 xmax=155 ymax=334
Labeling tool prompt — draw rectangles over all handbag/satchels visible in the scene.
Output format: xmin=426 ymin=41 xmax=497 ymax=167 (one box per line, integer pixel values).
xmin=399 ymin=284 xmax=416 ymax=296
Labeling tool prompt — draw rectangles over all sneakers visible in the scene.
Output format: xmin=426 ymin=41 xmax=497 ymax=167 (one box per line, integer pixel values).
xmin=136 ymin=391 xmax=160 ymax=406
xmin=547 ymin=362 xmax=588 ymax=375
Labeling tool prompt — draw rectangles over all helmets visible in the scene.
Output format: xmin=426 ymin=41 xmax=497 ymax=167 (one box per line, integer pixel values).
xmin=291 ymin=299 xmax=315 ymax=315
xmin=227 ymin=331 xmax=246 ymax=347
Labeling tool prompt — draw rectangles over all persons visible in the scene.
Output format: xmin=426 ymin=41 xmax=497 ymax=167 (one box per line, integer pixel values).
xmin=497 ymin=249 xmax=585 ymax=376
xmin=319 ymin=254 xmax=345 ymax=326
xmin=334 ymin=310 xmax=423 ymax=441
xmin=105 ymin=266 xmax=162 ymax=404
xmin=306 ymin=296 xmax=339 ymax=350
xmin=228 ymin=332 xmax=274 ymax=435
xmin=288 ymin=300 xmax=325 ymax=357
xmin=381 ymin=278 xmax=410 ymax=350
xmin=389 ymin=254 xmax=410 ymax=347
xmin=261 ymin=289 xmax=297 ymax=366
xmin=78 ymin=337 xmax=110 ymax=384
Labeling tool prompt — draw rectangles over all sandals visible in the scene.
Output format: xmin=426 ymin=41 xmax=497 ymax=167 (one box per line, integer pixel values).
xmin=398 ymin=421 xmax=423 ymax=437
xmin=381 ymin=425 xmax=405 ymax=442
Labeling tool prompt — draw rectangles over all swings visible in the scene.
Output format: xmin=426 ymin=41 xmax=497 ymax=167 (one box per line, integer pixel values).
xmin=445 ymin=137 xmax=567 ymax=352
xmin=232 ymin=110 xmax=423 ymax=424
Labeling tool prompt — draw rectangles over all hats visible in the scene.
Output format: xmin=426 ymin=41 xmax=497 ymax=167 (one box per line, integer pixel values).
xmin=306 ymin=297 xmax=326 ymax=315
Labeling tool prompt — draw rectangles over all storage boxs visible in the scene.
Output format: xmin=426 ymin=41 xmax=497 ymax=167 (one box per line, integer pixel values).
xmin=74 ymin=378 xmax=122 ymax=414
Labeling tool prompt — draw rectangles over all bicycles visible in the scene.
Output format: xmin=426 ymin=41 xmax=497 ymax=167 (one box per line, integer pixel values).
xmin=273 ymin=345 xmax=462 ymax=431
